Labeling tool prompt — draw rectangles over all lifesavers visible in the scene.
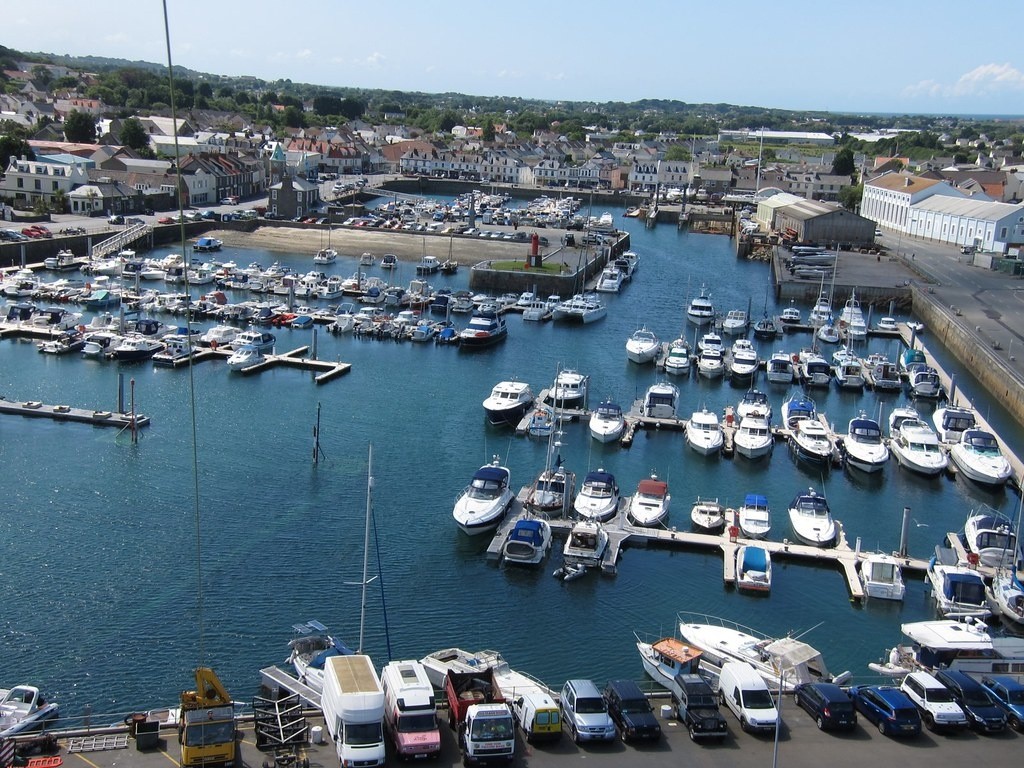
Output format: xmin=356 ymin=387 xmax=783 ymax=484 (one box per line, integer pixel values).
xmin=792 ymin=355 xmax=799 ymax=360
xmin=967 ymin=553 xmax=978 ymax=564
xmin=729 ymin=526 xmax=739 ymax=537
xmin=876 ymin=367 xmax=882 ymax=373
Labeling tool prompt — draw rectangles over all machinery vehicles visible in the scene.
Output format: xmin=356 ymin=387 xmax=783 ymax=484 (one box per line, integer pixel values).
xmin=177 ymin=667 xmax=239 ymax=768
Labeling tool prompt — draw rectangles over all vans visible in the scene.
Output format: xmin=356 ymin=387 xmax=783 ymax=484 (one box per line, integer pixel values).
xmin=718 ymin=662 xmax=781 ymax=734
xmin=560 ymin=679 xmax=616 ymax=746
xmin=514 ymin=693 xmax=563 ymax=744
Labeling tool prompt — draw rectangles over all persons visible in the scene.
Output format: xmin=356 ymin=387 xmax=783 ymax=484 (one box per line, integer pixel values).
xmin=514 ymin=221 xmax=518 ymax=230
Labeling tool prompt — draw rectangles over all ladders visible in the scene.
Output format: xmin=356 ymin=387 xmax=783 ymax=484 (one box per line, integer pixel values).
xmin=66 ymin=736 xmax=130 ymax=754
xmin=26 ymin=757 xmax=63 ymax=768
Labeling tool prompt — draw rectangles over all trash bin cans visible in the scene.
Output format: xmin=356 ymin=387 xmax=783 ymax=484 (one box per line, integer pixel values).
xmin=311 ymin=726 xmax=324 ymax=743
xmin=134 ymin=720 xmax=160 ymax=750
xmin=661 ymin=705 xmax=672 ymax=718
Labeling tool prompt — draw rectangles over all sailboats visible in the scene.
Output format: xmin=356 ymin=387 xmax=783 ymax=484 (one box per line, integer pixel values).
xmin=286 ymin=436 xmax=377 ymax=693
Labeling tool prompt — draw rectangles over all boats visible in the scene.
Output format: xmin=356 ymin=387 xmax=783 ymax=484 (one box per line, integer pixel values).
xmin=0 ymin=684 xmax=59 ymax=737
xmin=632 ymin=630 xmax=722 ymax=693
xmin=1 ymin=186 xmax=1024 ymax=630
xmin=867 ymin=619 xmax=1024 ymax=687
xmin=417 ymin=647 xmax=551 ymax=704
xmin=677 ymin=610 xmax=837 ymax=690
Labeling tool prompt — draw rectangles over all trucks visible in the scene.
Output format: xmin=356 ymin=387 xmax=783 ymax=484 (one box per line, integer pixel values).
xmin=446 ymin=667 xmax=515 ymax=767
xmin=380 ymin=660 xmax=442 ymax=763
xmin=321 ymin=655 xmax=388 ymax=768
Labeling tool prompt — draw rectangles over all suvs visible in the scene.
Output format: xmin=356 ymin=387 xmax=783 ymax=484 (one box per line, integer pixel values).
xmin=900 ymin=671 xmax=967 ymax=734
xmin=670 ymin=674 xmax=728 ymax=741
xmin=934 ymin=670 xmax=1008 ymax=736
xmin=847 ymin=683 xmax=922 ymax=738
xmin=792 ymin=682 xmax=858 ymax=731
xmin=602 ymin=678 xmax=661 ymax=743
xmin=980 ymin=673 xmax=1024 ymax=732
